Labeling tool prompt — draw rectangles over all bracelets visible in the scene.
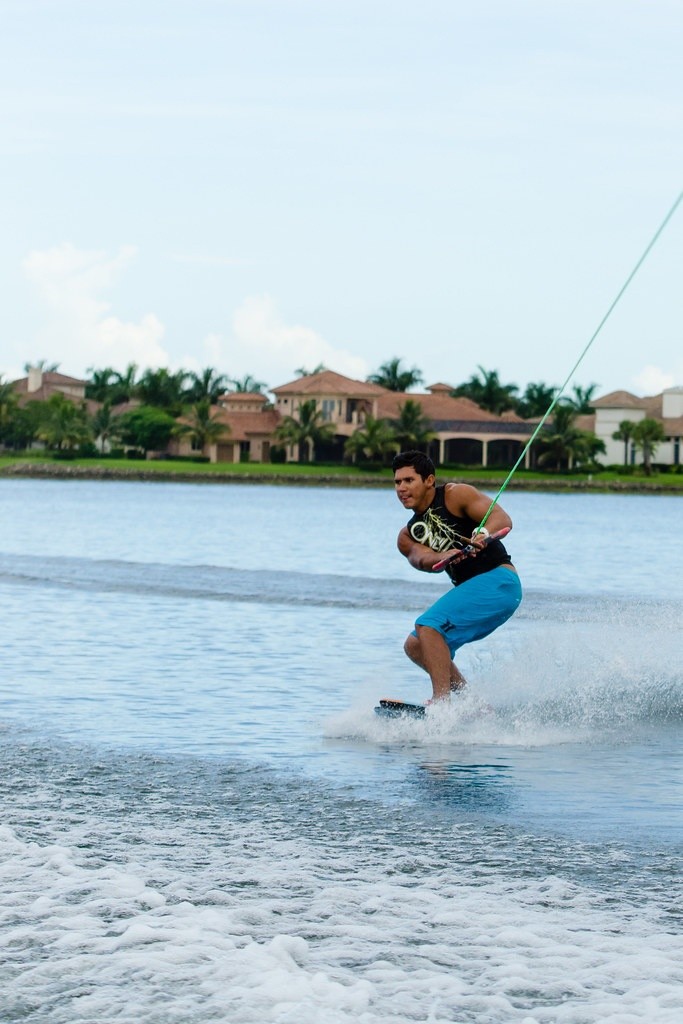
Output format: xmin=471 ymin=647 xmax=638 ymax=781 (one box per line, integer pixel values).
xmin=472 ymin=526 xmax=490 ymax=538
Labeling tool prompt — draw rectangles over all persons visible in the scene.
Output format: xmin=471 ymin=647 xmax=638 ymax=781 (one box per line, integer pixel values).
xmin=392 ymin=450 xmax=523 ymax=712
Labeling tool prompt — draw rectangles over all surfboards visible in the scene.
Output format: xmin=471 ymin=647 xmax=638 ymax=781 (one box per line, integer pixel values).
xmin=373 ymin=698 xmax=427 ymax=720
